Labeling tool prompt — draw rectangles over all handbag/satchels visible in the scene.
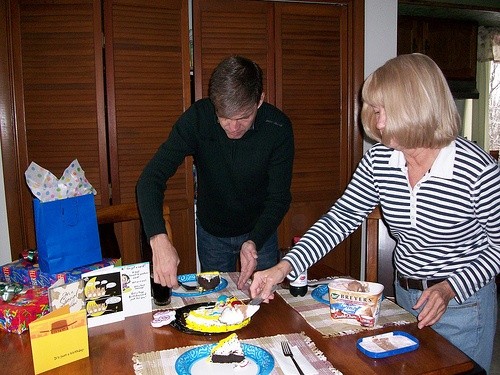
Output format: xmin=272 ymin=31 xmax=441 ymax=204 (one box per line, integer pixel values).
xmin=33 ymin=194 xmax=102 ymax=274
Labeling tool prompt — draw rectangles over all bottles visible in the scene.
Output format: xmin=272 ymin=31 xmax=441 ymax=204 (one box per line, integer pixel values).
xmin=289 ymin=237 xmax=307 ymax=296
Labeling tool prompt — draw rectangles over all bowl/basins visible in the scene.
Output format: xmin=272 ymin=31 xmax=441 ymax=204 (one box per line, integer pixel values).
xmin=327 ymin=278 xmax=384 ymax=329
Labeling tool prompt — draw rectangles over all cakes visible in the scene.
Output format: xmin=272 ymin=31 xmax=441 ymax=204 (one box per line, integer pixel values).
xmin=185 ymin=296 xmax=252 ymax=333
xmin=208 ymin=332 xmax=249 ymax=367
xmin=196 ymin=271 xmax=221 ymax=291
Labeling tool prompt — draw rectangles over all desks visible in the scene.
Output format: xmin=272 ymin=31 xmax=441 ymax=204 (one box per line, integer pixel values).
xmin=0 ymin=260 xmax=487 ymax=375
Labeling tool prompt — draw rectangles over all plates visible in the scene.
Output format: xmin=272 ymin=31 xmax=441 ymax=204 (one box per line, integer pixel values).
xmin=169 ymin=301 xmax=253 ymax=336
xmin=312 ymin=285 xmax=330 ymax=303
xmin=171 ymin=273 xmax=228 ymax=297
xmin=175 ymin=342 xmax=274 ymax=375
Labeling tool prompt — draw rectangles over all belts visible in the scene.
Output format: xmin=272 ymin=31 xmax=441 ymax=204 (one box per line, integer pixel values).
xmin=396 ymin=271 xmax=448 ymax=291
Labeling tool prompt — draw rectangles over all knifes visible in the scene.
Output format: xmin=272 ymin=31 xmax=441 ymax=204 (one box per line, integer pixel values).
xmin=248 ymin=285 xmax=279 ymax=305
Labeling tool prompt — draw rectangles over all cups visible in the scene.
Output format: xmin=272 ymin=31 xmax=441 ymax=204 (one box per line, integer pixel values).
xmin=150 ymin=265 xmax=171 ymax=305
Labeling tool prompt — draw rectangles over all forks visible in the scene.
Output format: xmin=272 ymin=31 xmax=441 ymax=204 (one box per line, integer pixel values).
xmin=281 ymin=342 xmax=305 ymax=375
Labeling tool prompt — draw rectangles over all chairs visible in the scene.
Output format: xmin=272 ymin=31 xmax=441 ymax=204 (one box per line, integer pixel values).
xmin=96 ymin=201 xmax=173 ymax=265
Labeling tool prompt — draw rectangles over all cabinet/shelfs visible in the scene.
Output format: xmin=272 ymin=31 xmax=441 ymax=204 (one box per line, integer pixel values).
xmin=400 ymin=16 xmax=478 ymax=100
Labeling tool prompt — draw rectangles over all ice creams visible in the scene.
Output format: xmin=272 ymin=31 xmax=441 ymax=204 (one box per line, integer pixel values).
xmin=329 ymin=280 xmax=366 ymax=293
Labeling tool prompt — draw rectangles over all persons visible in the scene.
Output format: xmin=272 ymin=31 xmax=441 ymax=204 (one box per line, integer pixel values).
xmin=249 ymin=53 xmax=500 ymax=375
xmin=135 ymin=54 xmax=295 ymax=291
